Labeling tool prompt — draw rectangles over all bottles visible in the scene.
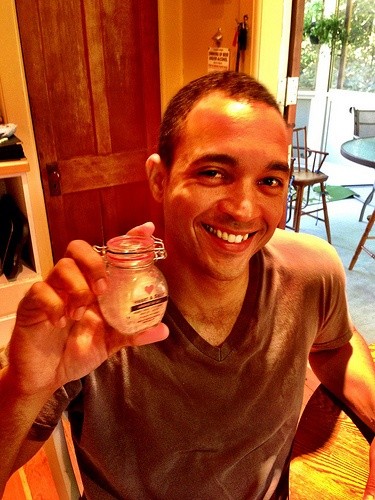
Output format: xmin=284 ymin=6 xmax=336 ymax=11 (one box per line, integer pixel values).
xmin=97 ymin=235 xmax=169 ymax=336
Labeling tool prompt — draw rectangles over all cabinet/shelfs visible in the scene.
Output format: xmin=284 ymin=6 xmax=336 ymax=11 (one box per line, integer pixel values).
xmin=1 ymin=1 xmax=87 ymax=500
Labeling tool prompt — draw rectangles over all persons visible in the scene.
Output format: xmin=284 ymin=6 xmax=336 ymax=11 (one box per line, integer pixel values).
xmin=0 ymin=70 xmax=375 ymax=500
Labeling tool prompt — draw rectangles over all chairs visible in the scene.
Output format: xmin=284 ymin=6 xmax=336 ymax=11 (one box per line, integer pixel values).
xmin=286 ymin=127 xmax=331 ymax=244
xmin=347 ymin=106 xmax=375 ymax=274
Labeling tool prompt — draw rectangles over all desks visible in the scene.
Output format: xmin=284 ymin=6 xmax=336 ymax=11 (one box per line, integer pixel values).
xmin=340 ymin=136 xmax=375 ymax=222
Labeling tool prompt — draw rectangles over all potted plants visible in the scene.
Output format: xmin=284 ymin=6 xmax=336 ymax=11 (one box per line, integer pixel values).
xmin=301 ymin=19 xmax=350 ymax=46
